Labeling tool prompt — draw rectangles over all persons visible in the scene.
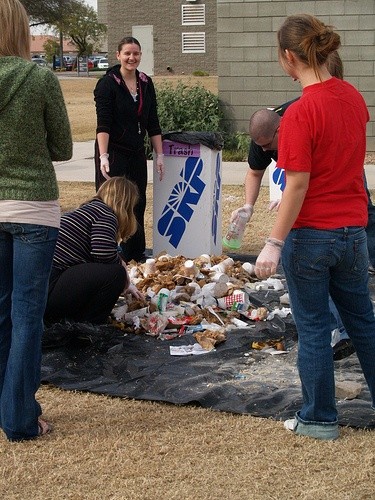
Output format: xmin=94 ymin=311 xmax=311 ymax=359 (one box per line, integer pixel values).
xmin=93 ymin=37 xmax=166 ymax=265
xmin=226 ymin=50 xmax=375 ymax=359
xmin=0 ymin=0 xmax=72 ymax=442
xmin=253 ymin=14 xmax=375 ymax=440
xmin=43 ymin=177 xmax=140 ymax=327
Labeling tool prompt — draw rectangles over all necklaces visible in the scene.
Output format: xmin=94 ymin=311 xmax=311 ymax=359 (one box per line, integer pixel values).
xmin=123 ymin=77 xmax=136 ymax=93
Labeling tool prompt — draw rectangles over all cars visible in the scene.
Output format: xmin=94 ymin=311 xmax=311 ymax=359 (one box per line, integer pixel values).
xmin=97 ymin=58 xmax=109 ymax=70
xmin=32 ymin=53 xmax=98 ymax=71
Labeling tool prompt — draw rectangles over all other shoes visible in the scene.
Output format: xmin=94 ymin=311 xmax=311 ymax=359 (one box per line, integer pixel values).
xmin=332 ymin=339 xmax=356 ymax=361
xmin=284 ymin=417 xmax=296 ymax=431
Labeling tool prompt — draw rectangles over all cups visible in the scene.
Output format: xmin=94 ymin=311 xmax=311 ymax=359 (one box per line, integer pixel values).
xmin=115 ymin=251 xmax=261 ymax=322
xmin=222 ymin=212 xmax=249 ymax=250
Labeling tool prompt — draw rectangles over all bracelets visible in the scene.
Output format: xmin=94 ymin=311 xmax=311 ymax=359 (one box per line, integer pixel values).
xmin=265 ymin=237 xmax=284 ymax=248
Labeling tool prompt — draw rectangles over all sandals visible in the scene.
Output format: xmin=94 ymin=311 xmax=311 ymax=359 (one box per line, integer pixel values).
xmin=35 ymin=421 xmax=55 ymax=437
